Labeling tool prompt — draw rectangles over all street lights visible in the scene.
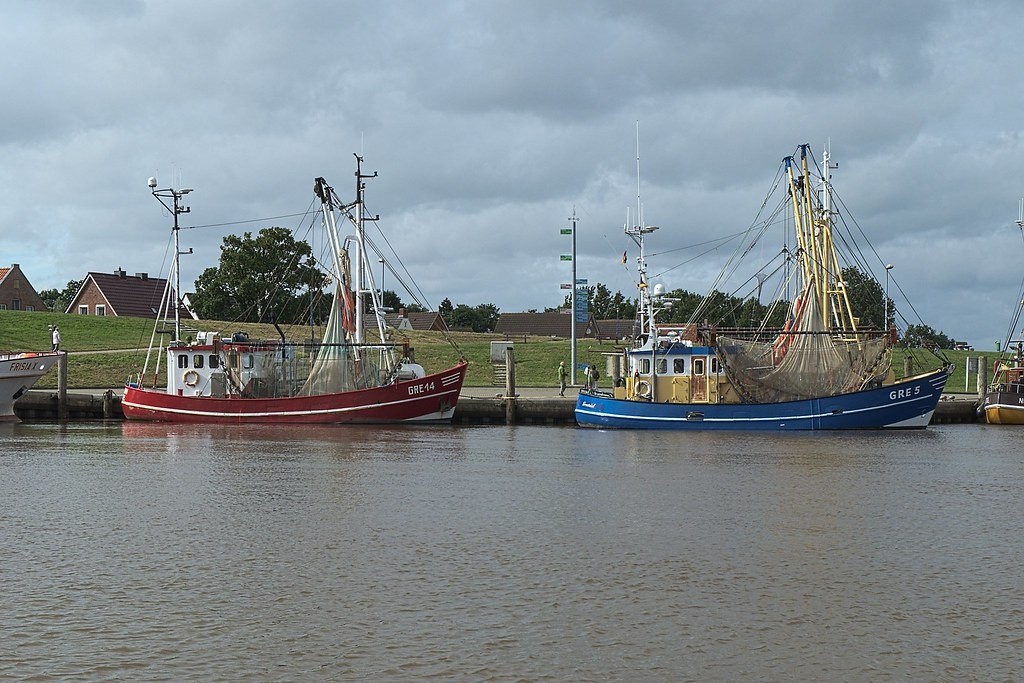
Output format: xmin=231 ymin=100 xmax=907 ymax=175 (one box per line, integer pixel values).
xmin=884 ymin=263 xmax=895 ymax=334
xmin=379 ymin=258 xmax=385 ymax=307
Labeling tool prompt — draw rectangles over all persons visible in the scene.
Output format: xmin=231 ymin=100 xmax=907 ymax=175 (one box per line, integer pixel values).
xmin=584 ymin=365 xmax=600 ymax=392
xmin=558 ymin=362 xmax=569 ymax=396
xmin=50 ymin=325 xmax=62 ymax=353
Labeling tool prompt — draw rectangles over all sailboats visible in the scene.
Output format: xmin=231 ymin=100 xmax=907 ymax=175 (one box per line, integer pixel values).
xmin=0 ymin=350 xmax=66 ymax=423
xmin=572 ymin=117 xmax=958 ymax=432
xmin=976 ymin=197 xmax=1024 ymax=427
xmin=118 ymin=152 xmax=470 ymax=428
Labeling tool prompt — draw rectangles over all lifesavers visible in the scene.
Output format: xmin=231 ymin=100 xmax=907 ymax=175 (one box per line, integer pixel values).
xmin=181 ymin=369 xmax=200 ymax=388
xmin=634 ymin=380 xmax=652 ymax=399
